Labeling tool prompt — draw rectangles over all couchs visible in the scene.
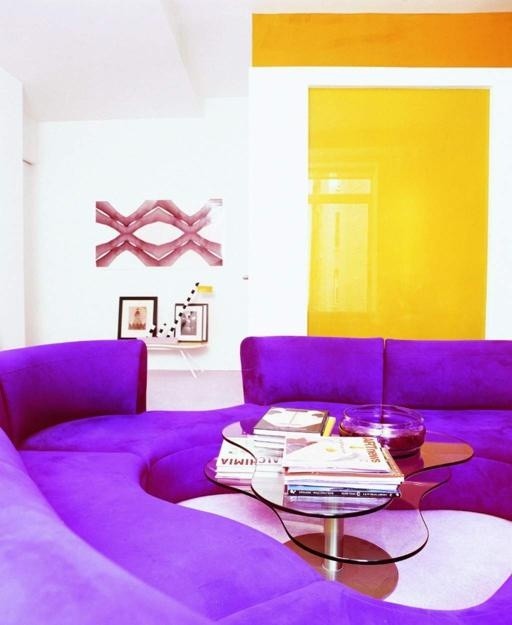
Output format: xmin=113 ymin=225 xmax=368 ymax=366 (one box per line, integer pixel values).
xmin=241 ymin=335 xmax=383 ymax=427
xmin=0 ymin=426 xmax=321 ymax=625
xmin=204 ymin=577 xmax=512 ymax=625
xmin=386 ymin=339 xmax=512 ymax=524
xmin=0 ymin=338 xmax=248 ymax=504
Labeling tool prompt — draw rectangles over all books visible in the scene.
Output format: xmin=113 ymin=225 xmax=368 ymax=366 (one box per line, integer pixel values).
xmin=215 ymin=432 xmax=284 ymax=481
xmin=282 ymin=435 xmax=403 ymax=501
xmin=253 ymin=407 xmax=337 ymax=436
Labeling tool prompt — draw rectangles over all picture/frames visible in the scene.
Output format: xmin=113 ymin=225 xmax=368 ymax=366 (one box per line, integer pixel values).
xmin=118 ymin=296 xmax=157 ymax=340
xmin=174 ymin=302 xmax=209 ymax=344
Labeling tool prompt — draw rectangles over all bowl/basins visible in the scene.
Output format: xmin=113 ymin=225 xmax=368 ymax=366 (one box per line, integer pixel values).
xmin=336 ymin=402 xmax=426 ymax=456
